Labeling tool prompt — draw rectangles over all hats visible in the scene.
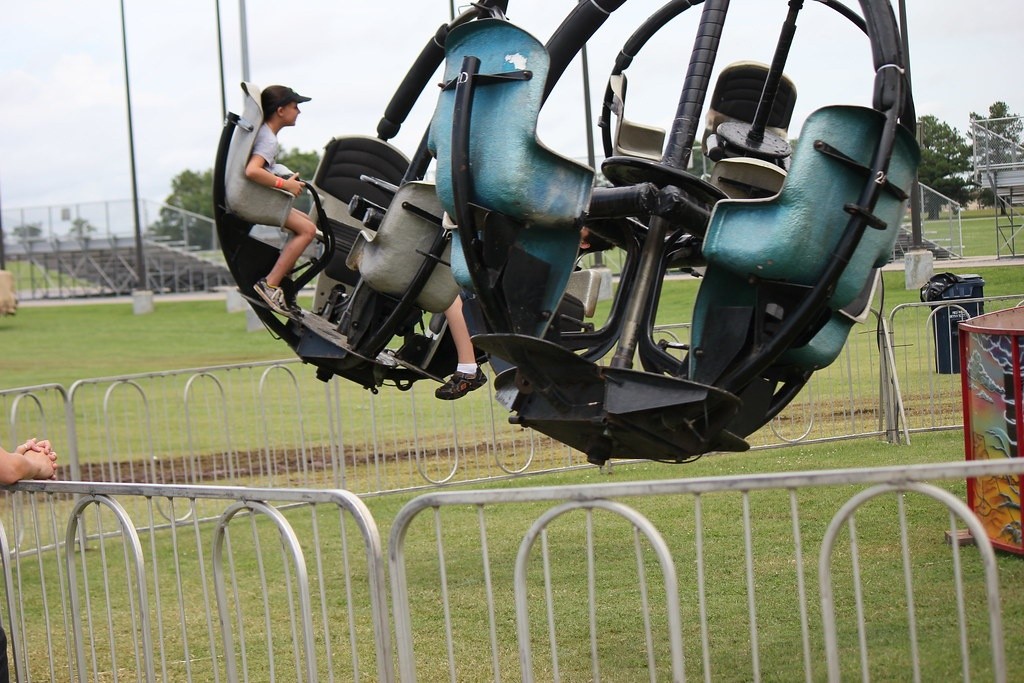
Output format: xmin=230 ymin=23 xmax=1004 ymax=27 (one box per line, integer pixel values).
xmin=261 ymin=85 xmax=312 ymax=111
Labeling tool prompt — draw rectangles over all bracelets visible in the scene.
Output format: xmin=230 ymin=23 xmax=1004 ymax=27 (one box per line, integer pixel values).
xmin=275 ymin=177 xmax=282 ymax=188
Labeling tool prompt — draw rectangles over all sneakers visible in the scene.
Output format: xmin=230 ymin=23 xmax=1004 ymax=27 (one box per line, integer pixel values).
xmin=254 ymin=277 xmax=290 ymax=316
xmin=434 ymin=363 xmax=487 ymax=400
xmin=286 ymin=294 xmax=301 ymax=312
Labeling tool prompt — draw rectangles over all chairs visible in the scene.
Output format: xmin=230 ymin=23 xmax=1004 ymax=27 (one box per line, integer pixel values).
xmin=224 ymin=80 xmax=293 ymax=235
xmin=426 ymin=18 xmax=922 ymax=385
xmin=307 ymin=135 xmax=462 ymax=350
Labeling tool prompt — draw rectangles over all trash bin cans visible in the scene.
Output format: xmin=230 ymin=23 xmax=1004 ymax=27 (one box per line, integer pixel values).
xmin=932 ymin=275 xmax=986 ymax=374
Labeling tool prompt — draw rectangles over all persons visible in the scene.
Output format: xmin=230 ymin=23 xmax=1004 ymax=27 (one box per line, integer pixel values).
xmin=245 ymin=85 xmax=316 ymax=317
xmin=435 ymin=227 xmax=616 ymax=401
xmin=0 ymin=438 xmax=57 ymax=683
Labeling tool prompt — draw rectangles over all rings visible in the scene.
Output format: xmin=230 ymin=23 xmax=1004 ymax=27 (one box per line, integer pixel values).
xmin=24 ymin=444 xmax=27 ymax=448
